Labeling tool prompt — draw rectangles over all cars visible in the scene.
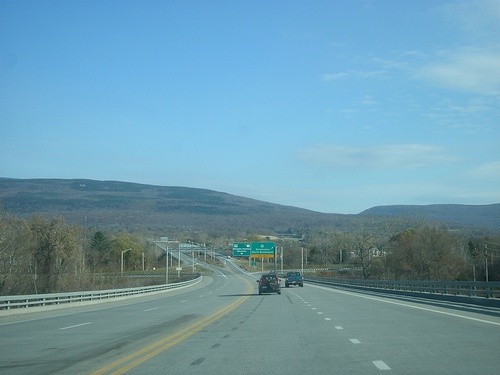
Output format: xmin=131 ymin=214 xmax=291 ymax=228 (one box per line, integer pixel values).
xmin=285 ymin=271 xmax=303 ymax=287
xmin=257 ymin=272 xmax=281 ymax=295
xmin=226 ymin=255 xmax=230 ymax=259
